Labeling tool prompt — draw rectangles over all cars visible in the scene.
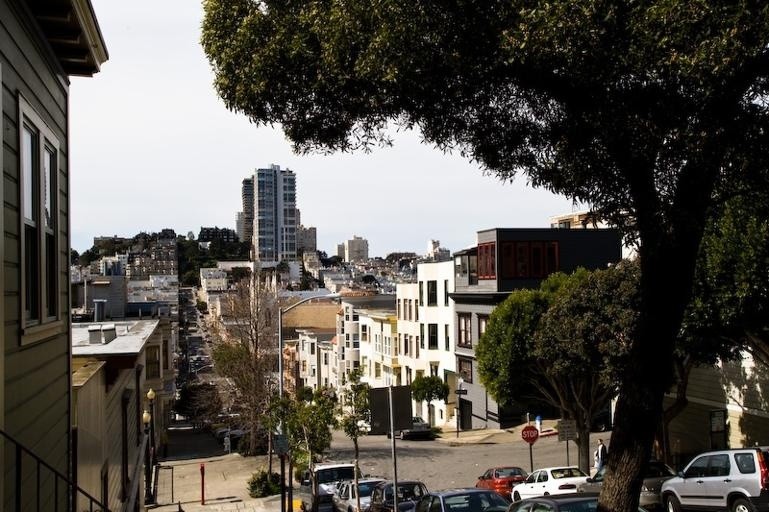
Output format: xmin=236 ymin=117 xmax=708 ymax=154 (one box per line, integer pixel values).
xmin=356 ymin=420 xmax=371 ymax=434
xmin=583 ymin=410 xmax=611 ymax=432
xmin=388 ymin=417 xmax=431 ymax=440
xmin=203 ymin=414 xmax=274 ymax=453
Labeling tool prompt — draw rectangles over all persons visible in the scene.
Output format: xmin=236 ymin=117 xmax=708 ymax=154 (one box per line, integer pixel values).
xmin=594 ymin=438 xmax=608 ymax=473
xmin=223 ymin=434 xmax=230 ymax=454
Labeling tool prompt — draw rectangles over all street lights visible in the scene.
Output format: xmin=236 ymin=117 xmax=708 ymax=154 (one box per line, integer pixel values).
xmin=278 ymin=293 xmax=342 ymax=435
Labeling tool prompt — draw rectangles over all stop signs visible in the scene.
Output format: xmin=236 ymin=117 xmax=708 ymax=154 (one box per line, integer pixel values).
xmin=522 ymin=425 xmax=538 ymax=443
